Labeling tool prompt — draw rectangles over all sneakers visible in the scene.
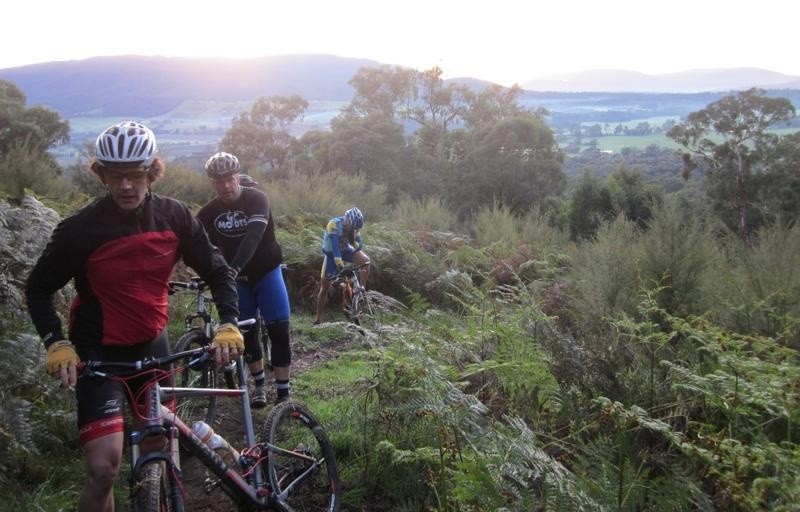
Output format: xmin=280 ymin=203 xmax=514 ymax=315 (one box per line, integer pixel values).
xmin=249 ymin=386 xmax=268 ymax=408
xmin=313 ymin=320 xmax=320 ymax=326
xmin=274 ymin=395 xmax=289 ymax=417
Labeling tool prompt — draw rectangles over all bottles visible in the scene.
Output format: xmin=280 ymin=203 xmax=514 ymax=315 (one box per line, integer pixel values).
xmin=189 ymin=421 xmax=242 ymax=468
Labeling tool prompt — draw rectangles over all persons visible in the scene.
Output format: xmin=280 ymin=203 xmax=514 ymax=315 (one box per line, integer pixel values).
xmin=194 ymin=152 xmax=292 ymax=409
xmin=313 ymin=207 xmax=370 ymax=325
xmin=22 ymin=121 xmax=245 ymax=512
xmin=238 ymin=174 xmax=258 ymax=188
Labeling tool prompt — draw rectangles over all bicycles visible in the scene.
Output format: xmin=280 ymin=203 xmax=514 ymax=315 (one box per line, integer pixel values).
xmin=160 ymin=271 xmax=252 ymax=453
xmin=54 ymin=326 xmax=348 ymax=510
xmin=330 ymin=258 xmax=384 ymax=342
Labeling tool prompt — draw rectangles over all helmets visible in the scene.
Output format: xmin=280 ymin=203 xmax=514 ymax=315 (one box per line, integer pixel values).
xmin=239 ymin=173 xmax=258 ymax=189
xmin=95 ymin=120 xmax=157 ymax=163
xmin=344 ymin=206 xmax=364 ymax=230
xmin=204 ymin=151 xmax=241 ymax=179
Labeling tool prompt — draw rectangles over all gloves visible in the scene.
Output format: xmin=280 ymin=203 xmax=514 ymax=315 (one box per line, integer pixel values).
xmin=213 ymin=323 xmax=245 ymax=353
xmin=44 ymin=340 xmax=80 ymax=374
